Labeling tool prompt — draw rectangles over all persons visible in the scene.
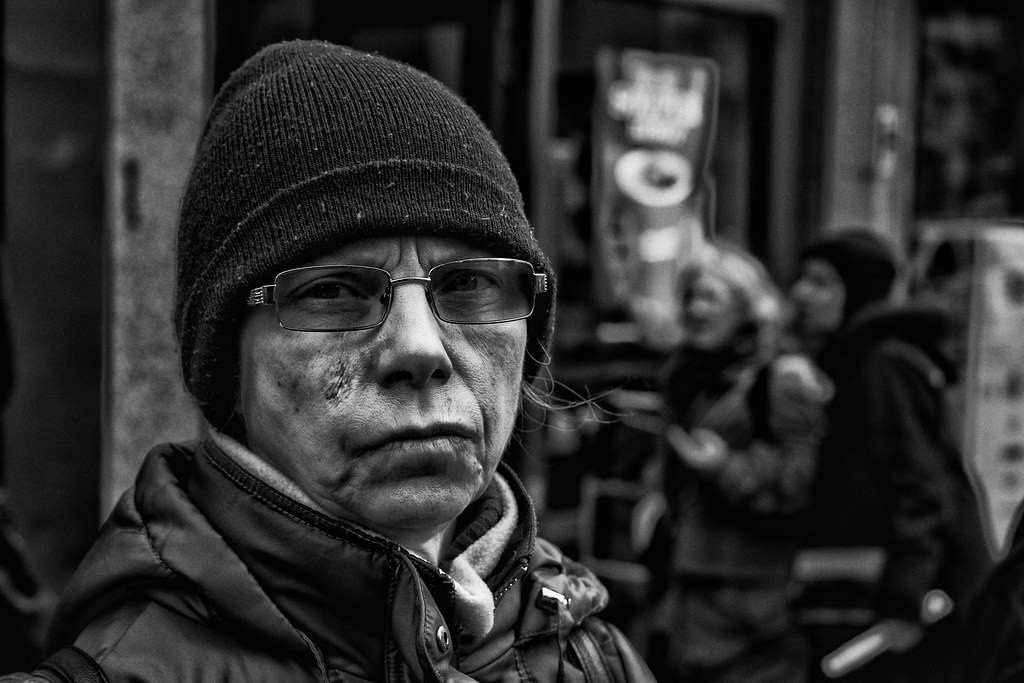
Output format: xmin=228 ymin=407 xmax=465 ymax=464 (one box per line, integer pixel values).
xmin=772 ymin=227 xmax=994 ymax=683
xmin=626 ymin=244 xmax=840 ymax=683
xmin=0 ymin=40 xmax=660 ymax=683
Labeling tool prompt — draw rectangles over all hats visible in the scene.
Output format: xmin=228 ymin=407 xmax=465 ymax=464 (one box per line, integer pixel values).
xmin=807 ymin=228 xmax=895 ymax=325
xmin=173 ymin=39 xmax=557 ymax=430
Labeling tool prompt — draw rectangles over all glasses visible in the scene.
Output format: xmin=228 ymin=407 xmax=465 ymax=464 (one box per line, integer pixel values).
xmin=247 ymin=256 xmax=548 ymax=334
